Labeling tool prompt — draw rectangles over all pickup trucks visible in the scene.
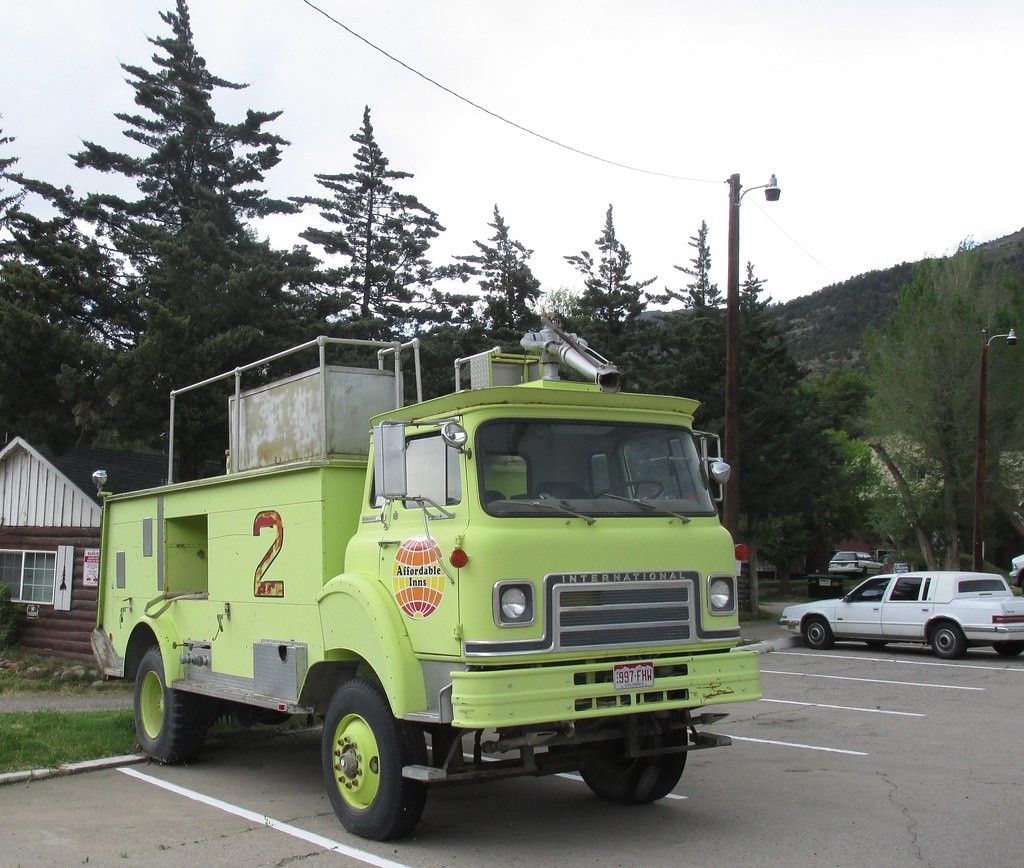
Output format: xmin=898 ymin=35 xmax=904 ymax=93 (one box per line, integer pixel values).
xmin=777 ymin=571 xmax=1024 ymax=659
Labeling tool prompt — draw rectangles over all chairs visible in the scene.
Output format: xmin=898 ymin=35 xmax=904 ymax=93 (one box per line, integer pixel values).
xmin=537 ymin=480 xmax=593 ymax=499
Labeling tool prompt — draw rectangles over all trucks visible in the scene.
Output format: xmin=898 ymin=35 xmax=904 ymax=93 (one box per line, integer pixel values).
xmin=89 ymin=310 xmax=763 ymax=842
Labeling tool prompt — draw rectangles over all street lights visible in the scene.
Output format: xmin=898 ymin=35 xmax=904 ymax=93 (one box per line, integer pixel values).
xmin=973 ymin=327 xmax=1019 ymax=573
xmin=726 ymin=174 xmax=781 ymax=546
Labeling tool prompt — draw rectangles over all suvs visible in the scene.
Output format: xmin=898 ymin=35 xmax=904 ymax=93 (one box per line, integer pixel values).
xmin=828 ymin=551 xmax=883 ymax=576
